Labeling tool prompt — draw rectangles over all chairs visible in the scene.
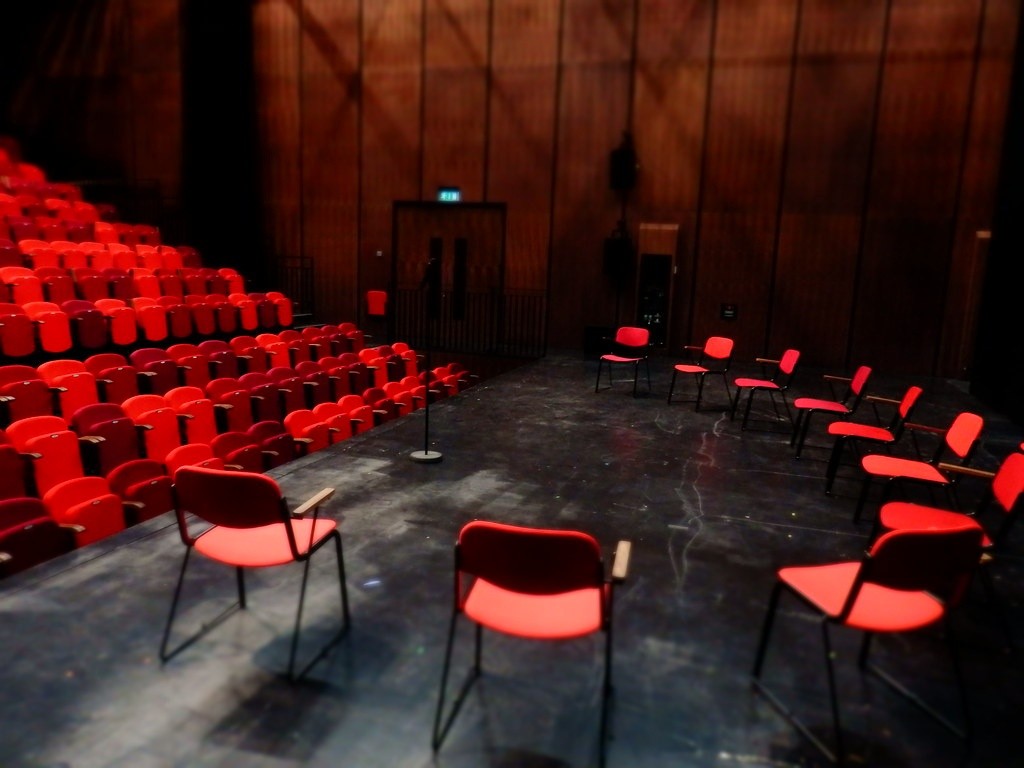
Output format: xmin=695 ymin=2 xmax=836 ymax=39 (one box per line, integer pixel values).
xmin=749 ymin=527 xmax=984 ymax=767
xmin=595 ymin=326 xmax=652 ymax=397
xmin=792 ymin=364 xmax=873 ymax=460
xmin=863 ymin=442 xmax=1024 ymax=555
xmin=852 ymin=411 xmax=985 ymax=523
xmin=432 ymin=520 xmax=632 ymax=768
xmin=0 ymin=144 xmax=479 ymax=578
xmin=824 ymin=385 xmax=923 ymax=496
xmin=668 ymin=335 xmax=734 ymax=413
xmin=160 ymin=463 xmax=350 ymax=690
xmin=730 ymin=348 xmax=800 ymax=435
